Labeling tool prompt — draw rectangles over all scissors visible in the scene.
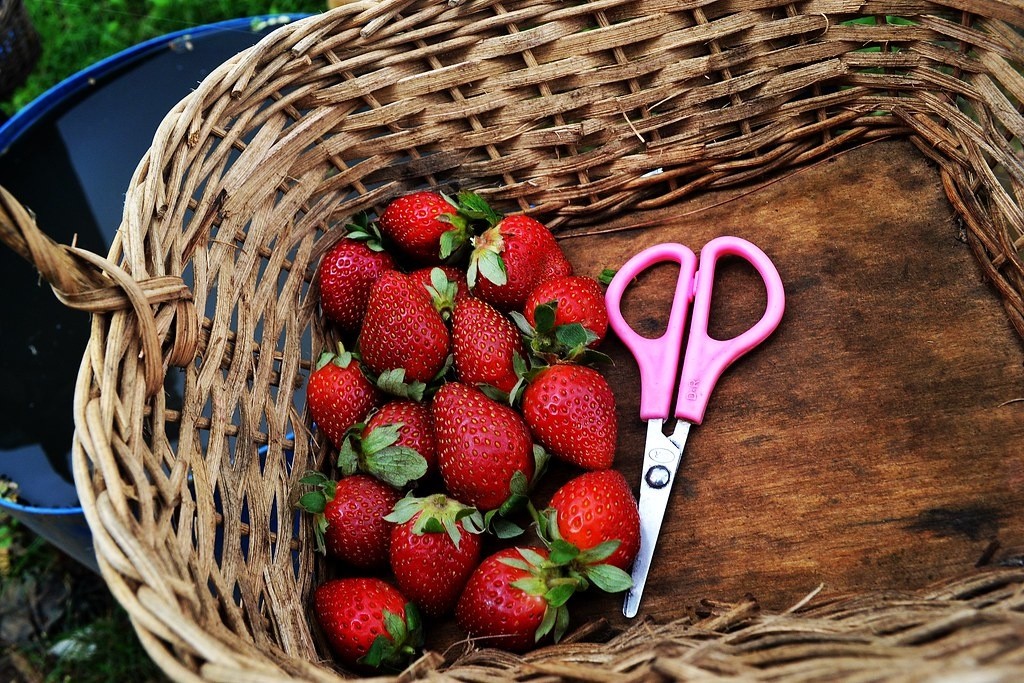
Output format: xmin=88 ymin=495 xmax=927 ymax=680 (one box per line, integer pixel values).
xmin=605 ymin=236 xmax=785 ymax=617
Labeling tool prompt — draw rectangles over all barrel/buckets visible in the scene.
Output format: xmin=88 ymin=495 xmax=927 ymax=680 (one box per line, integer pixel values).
xmin=0 ymin=15 xmax=315 ymax=580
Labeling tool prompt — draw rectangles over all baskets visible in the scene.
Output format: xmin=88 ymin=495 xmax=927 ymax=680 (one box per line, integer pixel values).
xmin=0 ymin=0 xmax=1024 ymax=683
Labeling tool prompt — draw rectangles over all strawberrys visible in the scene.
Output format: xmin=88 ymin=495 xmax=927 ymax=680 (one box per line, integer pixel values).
xmin=308 ymin=194 xmax=641 ymax=667
